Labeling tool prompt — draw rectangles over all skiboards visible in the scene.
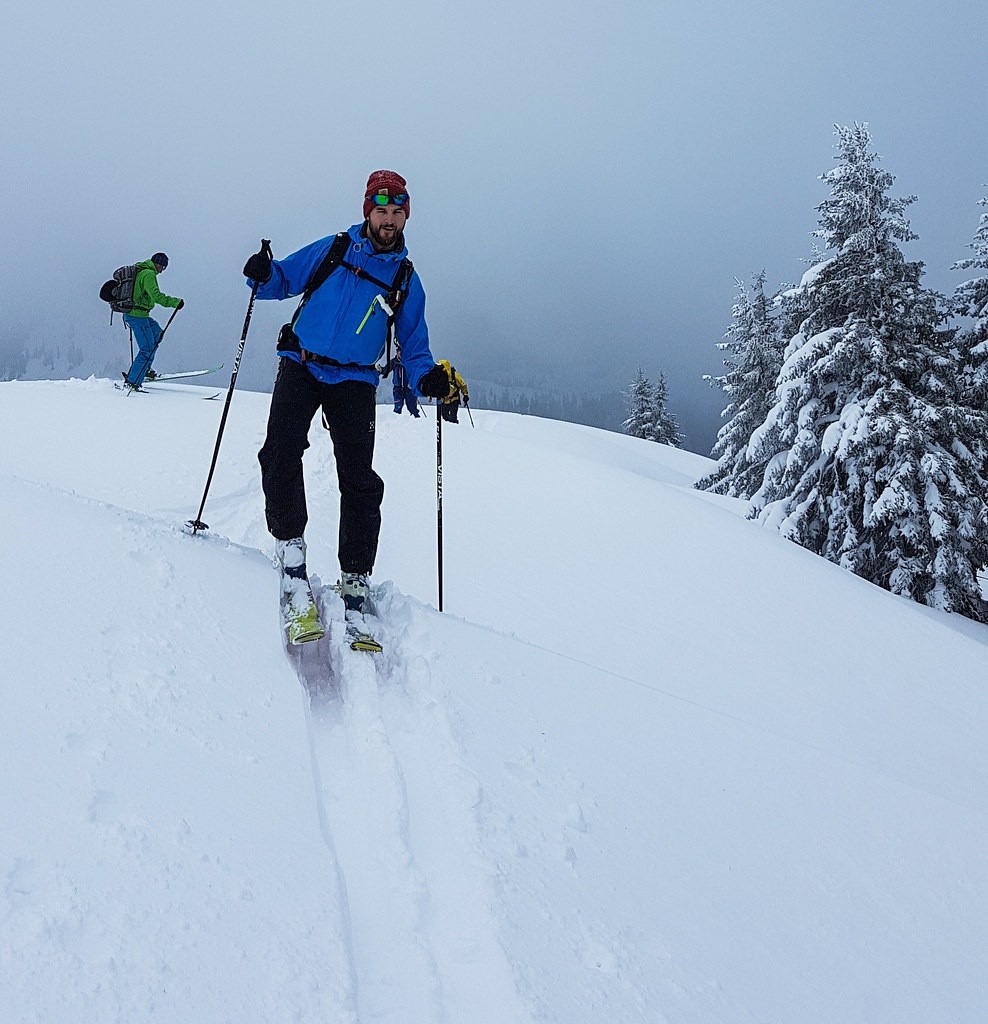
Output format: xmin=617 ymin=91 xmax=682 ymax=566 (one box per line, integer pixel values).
xmin=114 ymin=360 xmax=225 ymax=400
xmin=274 ymin=550 xmax=384 ymax=652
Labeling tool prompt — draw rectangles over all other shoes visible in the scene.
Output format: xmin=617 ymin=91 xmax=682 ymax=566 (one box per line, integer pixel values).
xmin=144 ymin=368 xmax=157 ymax=378
xmin=121 ymin=371 xmax=146 ymax=392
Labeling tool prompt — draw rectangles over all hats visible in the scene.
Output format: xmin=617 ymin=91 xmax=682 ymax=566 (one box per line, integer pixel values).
xmin=363 ymin=169 xmax=411 ymax=220
xmin=151 ymin=252 xmax=168 ymax=266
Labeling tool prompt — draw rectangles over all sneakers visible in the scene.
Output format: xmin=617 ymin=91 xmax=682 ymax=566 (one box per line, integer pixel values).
xmin=340 ymin=570 xmax=370 ymax=610
xmin=274 ymin=534 xmax=307 ymax=580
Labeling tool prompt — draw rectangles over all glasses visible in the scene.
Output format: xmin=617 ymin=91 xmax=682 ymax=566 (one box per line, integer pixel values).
xmin=163 ymin=266 xmax=166 ymax=270
xmin=365 ymin=193 xmax=409 ymax=206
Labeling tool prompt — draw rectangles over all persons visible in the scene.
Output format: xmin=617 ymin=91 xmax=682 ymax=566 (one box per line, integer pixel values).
xmin=376 ymin=348 xmax=420 ymax=418
xmin=243 ymin=169 xmax=451 ymax=607
xmin=437 ymin=360 xmax=470 ymax=423
xmin=122 ymin=253 xmax=184 ymax=393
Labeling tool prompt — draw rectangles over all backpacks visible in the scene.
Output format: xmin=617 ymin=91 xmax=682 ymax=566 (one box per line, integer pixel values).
xmin=100 ymin=265 xmax=149 ymax=313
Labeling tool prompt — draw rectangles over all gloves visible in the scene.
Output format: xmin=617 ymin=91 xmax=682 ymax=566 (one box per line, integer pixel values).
xmin=176 ymin=300 xmax=184 ymax=310
xmin=243 ymin=252 xmax=270 ymax=280
xmin=417 ymin=364 xmax=450 ymax=400
xmin=376 ymin=364 xmax=382 ymax=372
xmin=463 ymin=395 xmax=469 ymax=402
